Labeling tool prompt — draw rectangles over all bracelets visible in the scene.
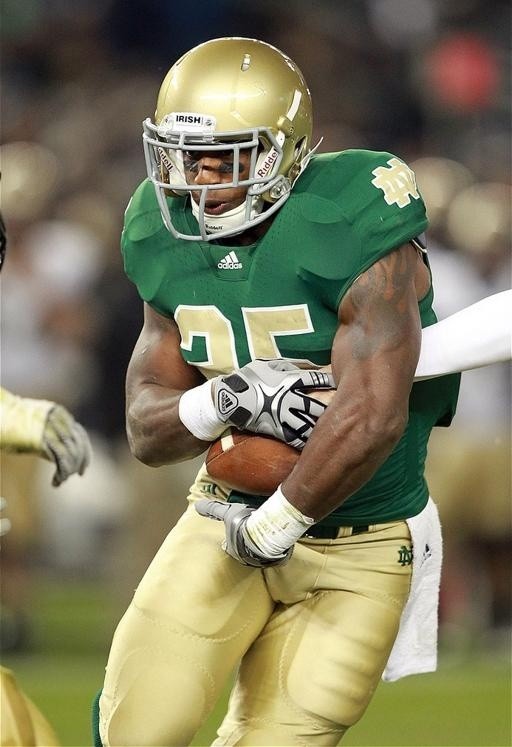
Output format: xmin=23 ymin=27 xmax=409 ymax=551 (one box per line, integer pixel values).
xmin=245 ymin=480 xmax=317 ymax=559
xmin=176 ymin=376 xmax=229 ymax=445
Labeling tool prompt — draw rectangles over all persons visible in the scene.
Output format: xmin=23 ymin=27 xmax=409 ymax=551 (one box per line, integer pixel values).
xmin=0 ymin=212 xmax=97 ymax=746
xmin=299 ymin=284 xmax=512 ymax=408
xmin=89 ymin=34 xmax=465 ymax=745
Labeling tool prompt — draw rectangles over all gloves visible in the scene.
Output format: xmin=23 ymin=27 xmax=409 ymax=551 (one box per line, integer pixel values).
xmin=214 ymin=357 xmax=337 ymax=453
xmin=40 ymin=404 xmax=93 ymax=488
xmin=195 ymin=499 xmax=296 ymax=569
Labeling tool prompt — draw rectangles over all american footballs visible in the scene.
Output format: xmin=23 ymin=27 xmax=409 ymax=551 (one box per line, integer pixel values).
xmin=205 ymin=389 xmax=341 ymax=499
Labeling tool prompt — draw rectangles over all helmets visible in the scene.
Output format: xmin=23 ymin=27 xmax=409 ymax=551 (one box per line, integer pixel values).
xmin=150 ymin=38 xmax=314 ymax=204
